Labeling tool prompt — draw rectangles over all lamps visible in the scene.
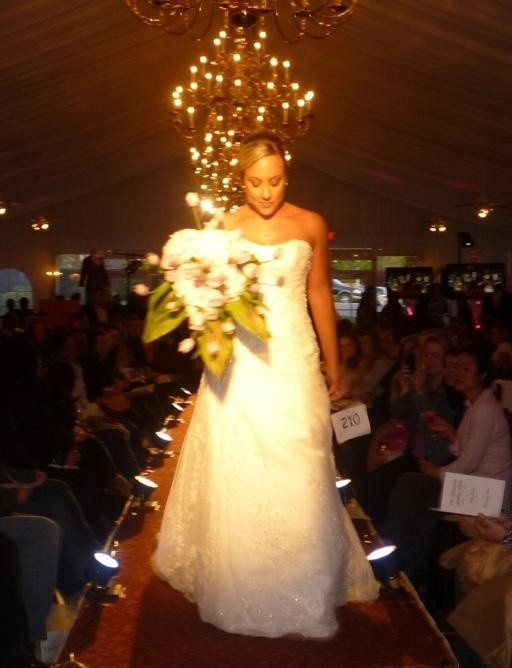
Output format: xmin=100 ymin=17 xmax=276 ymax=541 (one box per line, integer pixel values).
xmin=426 ymin=220 xmax=447 ymax=232
xmin=123 ymin=0 xmax=360 ymax=227
xmin=458 ymin=232 xmax=474 ymax=248
xmin=477 ymin=207 xmax=494 ymax=219
xmin=368 ymin=544 xmax=402 ymax=593
xmin=30 ymin=216 xmax=50 ymax=232
xmin=45 ymin=267 xmax=63 ymax=277
xmin=334 ymin=478 xmax=353 ymax=505
xmin=90 ymin=386 xmax=192 ymax=596
xmin=0 ymin=201 xmax=6 ymax=215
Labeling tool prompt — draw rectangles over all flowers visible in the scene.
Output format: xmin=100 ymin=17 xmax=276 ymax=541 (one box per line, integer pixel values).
xmin=129 ymin=229 xmax=270 ymax=379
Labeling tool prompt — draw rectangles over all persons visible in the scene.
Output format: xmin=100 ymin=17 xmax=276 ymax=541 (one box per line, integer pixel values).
xmin=152 ymin=133 xmax=382 ymax=638
xmin=1 ymin=250 xmax=182 ymax=667
xmin=336 ymin=278 xmax=511 ymax=667
